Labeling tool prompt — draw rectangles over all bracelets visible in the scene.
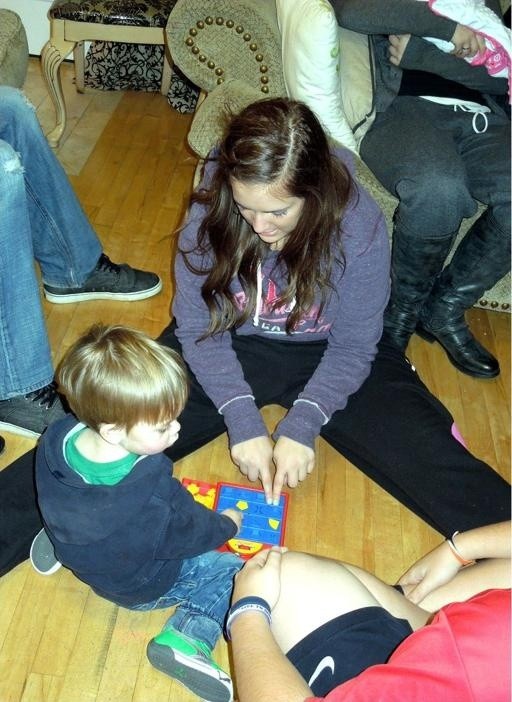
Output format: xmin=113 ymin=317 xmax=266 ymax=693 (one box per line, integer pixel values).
xmin=445 ymin=531 xmax=477 ymax=570
xmin=223 ymin=596 xmax=274 ymax=642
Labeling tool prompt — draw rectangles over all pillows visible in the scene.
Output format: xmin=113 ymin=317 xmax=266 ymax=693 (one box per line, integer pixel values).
xmin=276 ymin=0 xmax=359 ymax=158
xmin=338 ymin=27 xmax=377 ymax=147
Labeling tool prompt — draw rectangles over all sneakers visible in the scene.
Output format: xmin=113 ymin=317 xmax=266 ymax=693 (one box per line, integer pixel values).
xmin=0 ymin=382 xmax=70 ymax=442
xmin=43 ymin=252 xmax=161 ymax=303
xmin=147 ymin=627 xmax=233 ymax=702
xmin=30 ymin=528 xmax=61 ymax=575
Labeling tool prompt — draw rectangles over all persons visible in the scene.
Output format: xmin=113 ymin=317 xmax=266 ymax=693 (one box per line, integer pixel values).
xmin=329 ymin=1 xmax=512 ymax=382
xmin=27 ymin=321 xmax=238 ymax=702
xmin=0 ymin=94 xmax=512 ymax=585
xmin=220 ymin=512 xmax=511 ymax=702
xmin=0 ymin=83 xmax=166 ymax=437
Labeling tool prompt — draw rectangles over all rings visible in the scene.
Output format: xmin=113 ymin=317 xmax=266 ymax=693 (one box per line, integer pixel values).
xmin=462 ymin=47 xmax=469 ymax=51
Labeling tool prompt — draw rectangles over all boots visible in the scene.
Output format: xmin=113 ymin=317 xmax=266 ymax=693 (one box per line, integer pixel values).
xmin=414 ymin=203 xmax=511 ymax=379
xmin=383 ymin=210 xmax=457 ymax=354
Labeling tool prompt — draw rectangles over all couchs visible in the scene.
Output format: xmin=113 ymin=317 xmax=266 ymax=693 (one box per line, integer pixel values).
xmin=166 ymin=0 xmax=510 ymax=314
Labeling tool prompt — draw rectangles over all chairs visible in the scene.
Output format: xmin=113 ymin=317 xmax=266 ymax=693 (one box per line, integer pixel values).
xmin=40 ymin=1 xmax=207 ymax=149
xmin=1 ymin=9 xmax=26 ymax=89
